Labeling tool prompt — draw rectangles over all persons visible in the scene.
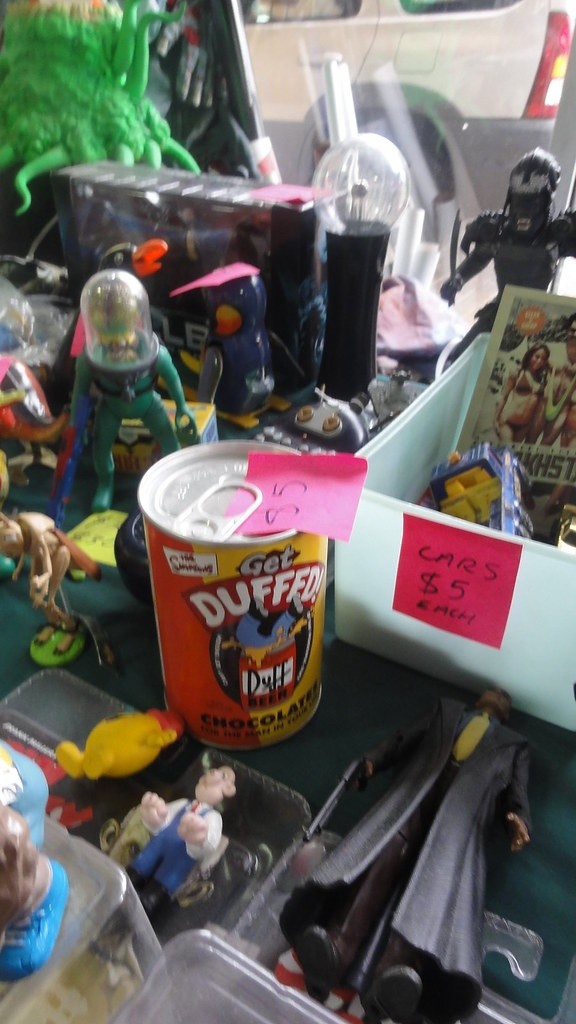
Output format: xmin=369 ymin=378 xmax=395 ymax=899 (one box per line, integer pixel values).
xmin=69 ymin=269 xmax=198 ymax=512
xmin=126 ymin=765 xmax=237 ymax=920
xmin=279 ymin=686 xmax=533 ymax=1024
xmin=493 ymin=315 xmax=576 ymax=447
xmin=0 ymin=512 xmax=77 ymax=655
xmin=440 ymin=147 xmax=576 ymax=369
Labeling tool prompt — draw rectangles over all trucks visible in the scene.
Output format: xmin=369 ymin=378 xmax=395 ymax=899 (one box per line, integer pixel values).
xmin=429 ymin=440 xmax=534 ymax=541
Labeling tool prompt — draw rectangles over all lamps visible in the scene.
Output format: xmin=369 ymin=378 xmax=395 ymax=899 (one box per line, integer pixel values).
xmin=312 ymin=133 xmax=412 ymax=400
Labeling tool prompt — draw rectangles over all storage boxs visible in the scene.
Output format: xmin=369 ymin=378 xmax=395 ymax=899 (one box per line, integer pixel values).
xmin=335 ymin=332 xmax=576 ymax=733
xmin=0 ymin=813 xmax=161 ymax=1024
xmin=112 ymin=399 xmax=220 ymax=473
xmin=51 ymin=160 xmax=343 ymax=396
xmin=106 ymin=930 xmax=350 ymax=1024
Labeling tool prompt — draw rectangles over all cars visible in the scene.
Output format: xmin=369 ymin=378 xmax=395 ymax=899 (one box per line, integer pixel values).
xmin=240 ymin=1 xmax=575 ymax=239
